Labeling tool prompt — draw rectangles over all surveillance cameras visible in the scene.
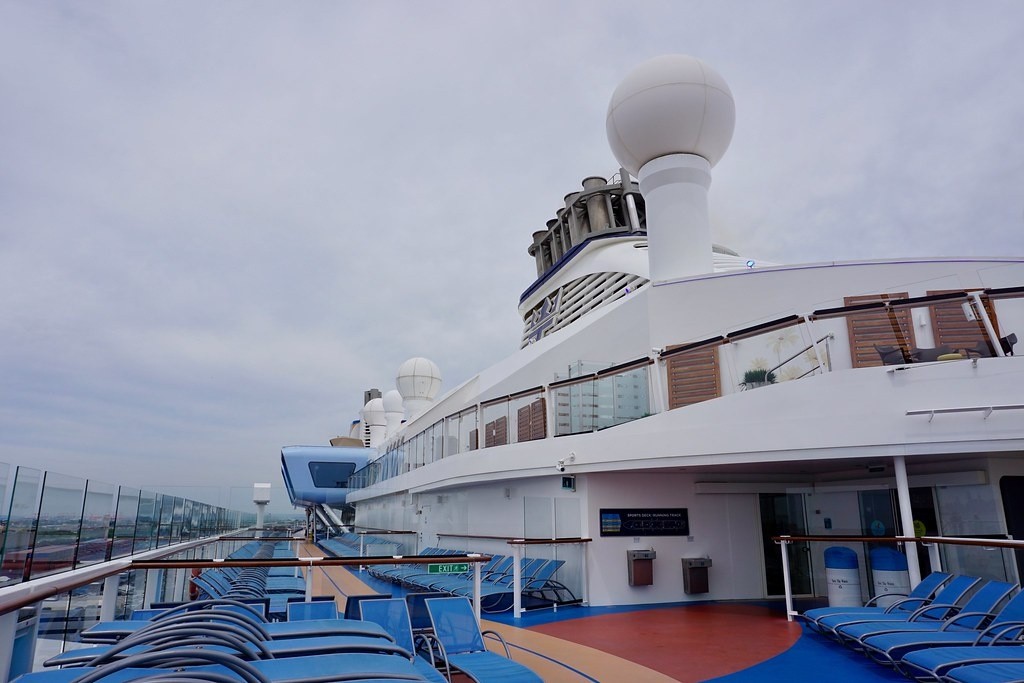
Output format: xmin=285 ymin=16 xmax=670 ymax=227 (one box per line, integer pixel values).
xmin=556 ymin=465 xmax=565 ymax=472
xmin=652 ymin=348 xmax=663 ymax=353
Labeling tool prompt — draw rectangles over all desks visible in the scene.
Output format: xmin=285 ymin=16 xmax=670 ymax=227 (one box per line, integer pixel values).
xmin=938 ymin=353 xmax=963 ymax=360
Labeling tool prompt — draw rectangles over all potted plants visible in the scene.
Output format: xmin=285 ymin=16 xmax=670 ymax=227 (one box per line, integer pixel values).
xmin=738 ymin=368 xmax=780 ymax=391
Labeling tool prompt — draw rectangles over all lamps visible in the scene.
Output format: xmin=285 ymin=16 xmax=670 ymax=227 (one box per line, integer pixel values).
xmin=919 ymin=313 xmax=927 ymax=327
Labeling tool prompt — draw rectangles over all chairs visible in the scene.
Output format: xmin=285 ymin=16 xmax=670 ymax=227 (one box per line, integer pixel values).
xmin=911 ymin=345 xmax=960 ymax=362
xmin=9 ymin=531 xmax=578 ymax=683
xmin=801 ymin=571 xmax=1024 ymax=683
xmin=964 ymin=333 xmax=1018 ymax=360
xmin=871 ymin=343 xmax=924 ymax=365
